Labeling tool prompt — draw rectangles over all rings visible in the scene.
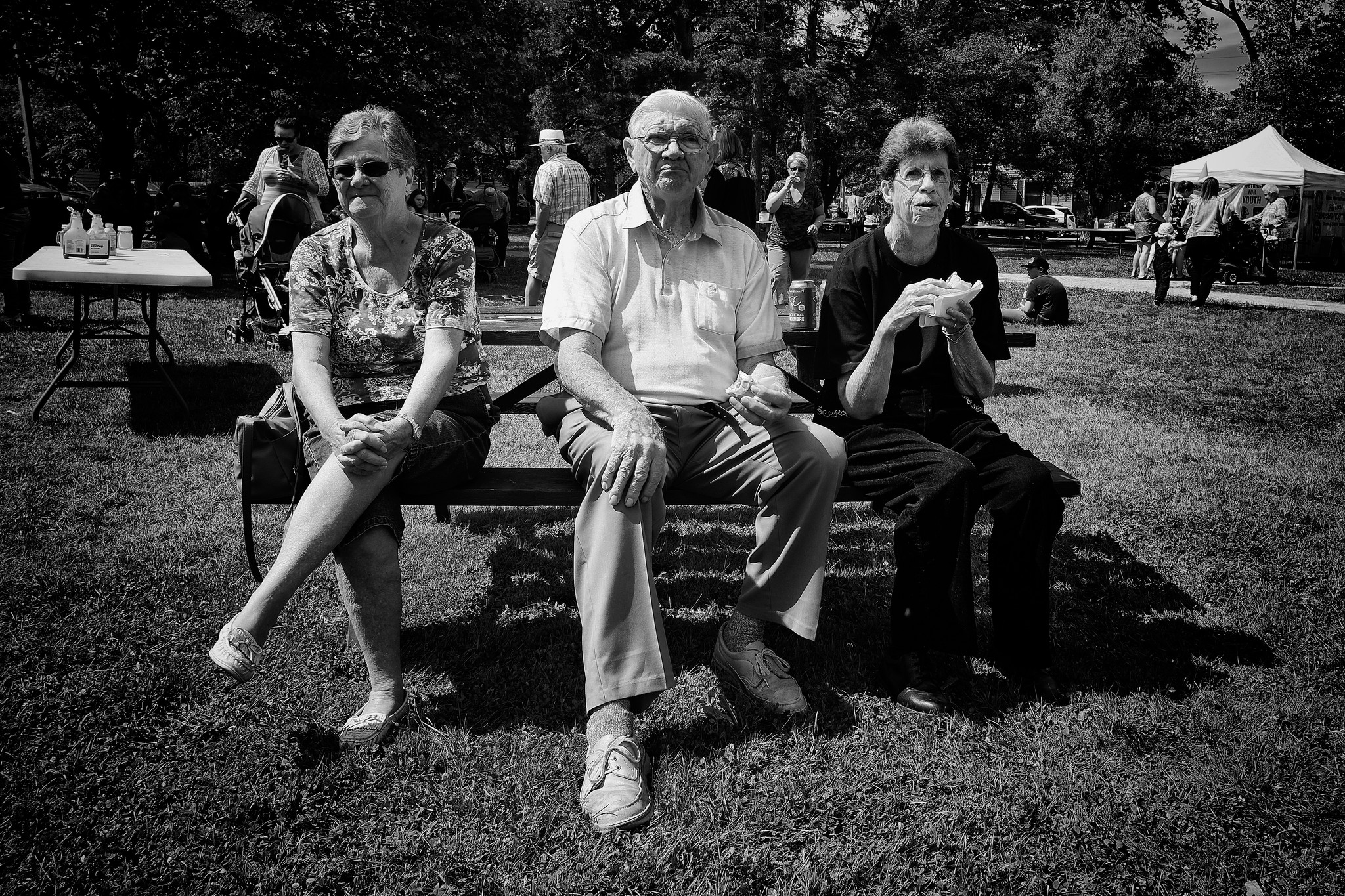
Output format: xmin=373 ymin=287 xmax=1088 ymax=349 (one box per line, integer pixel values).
xmin=948 ymin=321 xmax=956 ymax=329
xmin=755 ymin=418 xmax=766 ymax=426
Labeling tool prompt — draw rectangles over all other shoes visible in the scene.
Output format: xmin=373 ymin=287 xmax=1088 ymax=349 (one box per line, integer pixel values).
xmin=1251 ymin=271 xmax=1266 ymax=277
xmin=1145 ymin=266 xmax=1156 ymax=279
xmin=1154 ymin=297 xmax=1164 ymax=307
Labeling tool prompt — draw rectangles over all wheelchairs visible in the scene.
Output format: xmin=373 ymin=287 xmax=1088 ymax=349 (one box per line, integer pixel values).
xmin=1187 ymin=220 xmax=1268 ymax=285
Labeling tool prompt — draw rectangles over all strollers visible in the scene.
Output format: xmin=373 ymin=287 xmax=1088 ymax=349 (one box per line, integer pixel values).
xmin=224 ymin=193 xmax=317 ymax=353
xmin=458 ymin=203 xmax=501 ymax=285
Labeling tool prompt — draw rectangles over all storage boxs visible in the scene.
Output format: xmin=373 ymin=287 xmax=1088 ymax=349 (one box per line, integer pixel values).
xmin=758 ymin=213 xmax=769 ymax=221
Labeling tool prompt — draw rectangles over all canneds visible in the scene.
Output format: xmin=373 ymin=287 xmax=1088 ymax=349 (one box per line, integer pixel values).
xmin=117 ymin=226 xmax=133 ymax=250
xmin=789 ymin=280 xmax=817 ymax=331
xmin=818 ymin=280 xmax=826 ymax=323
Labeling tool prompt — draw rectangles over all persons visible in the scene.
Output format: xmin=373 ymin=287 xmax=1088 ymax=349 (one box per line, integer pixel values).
xmin=813 ymin=118 xmax=1065 ymax=713
xmin=948 ymin=190 xmax=967 ymax=232
xmin=1130 ymin=176 xmax=1288 ymax=308
xmin=524 ymin=129 xmax=592 ymax=307
xmin=846 ymin=186 xmax=867 ymax=243
xmin=1000 ymin=257 xmax=1069 ymax=327
xmin=208 ymin=106 xmax=492 ymax=749
xmin=225 ymin=117 xmax=511 ymax=268
xmin=697 ymin=130 xmax=756 ymax=232
xmin=765 ymin=153 xmax=825 ymax=310
xmin=538 ymin=89 xmax=848 ymax=833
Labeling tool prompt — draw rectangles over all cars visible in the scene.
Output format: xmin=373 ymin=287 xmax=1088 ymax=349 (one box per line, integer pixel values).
xmin=1099 ymin=212 xmax=1136 ymax=242
xmin=945 ymin=211 xmax=988 ymax=239
xmin=0 ymin=166 xmax=95 ymax=235
xmin=131 ymin=181 xmax=162 ymax=196
xmin=500 ymin=190 xmax=531 ymax=225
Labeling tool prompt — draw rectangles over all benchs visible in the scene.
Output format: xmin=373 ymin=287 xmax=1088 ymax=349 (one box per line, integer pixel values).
xmin=244 ymin=460 xmax=1083 ymax=504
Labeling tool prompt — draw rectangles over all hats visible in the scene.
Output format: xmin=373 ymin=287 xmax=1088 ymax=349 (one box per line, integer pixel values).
xmin=483 ymin=183 xmax=497 ymax=202
xmin=1154 ymin=222 xmax=1178 ymax=239
xmin=528 ymin=129 xmax=577 ymax=146
xmin=445 ymin=163 xmax=457 ymax=169
xmin=1020 ymin=256 xmax=1050 ymax=272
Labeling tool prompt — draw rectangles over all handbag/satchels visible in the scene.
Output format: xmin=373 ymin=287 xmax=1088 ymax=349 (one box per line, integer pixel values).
xmin=229 ymin=382 xmax=310 ymax=506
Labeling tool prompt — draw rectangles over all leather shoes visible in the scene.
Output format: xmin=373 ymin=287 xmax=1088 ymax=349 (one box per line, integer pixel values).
xmin=337 ymin=687 xmax=410 ymax=751
xmin=1021 ymin=668 xmax=1069 ymax=706
xmin=712 ymin=621 xmax=811 ymax=722
xmin=580 ymin=734 xmax=655 ymax=833
xmin=895 ymin=679 xmax=951 ymax=718
xmin=208 ymin=611 xmax=264 ymax=683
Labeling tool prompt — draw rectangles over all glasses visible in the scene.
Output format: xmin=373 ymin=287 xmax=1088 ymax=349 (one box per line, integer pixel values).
xmin=632 ymin=132 xmax=710 ymax=153
xmin=538 ymin=147 xmax=544 ymax=154
xmin=895 ymin=166 xmax=957 ymax=183
xmin=274 ymin=134 xmax=296 ymax=143
xmin=327 ymin=160 xmax=399 ymax=180
xmin=789 ymin=166 xmax=806 ymax=172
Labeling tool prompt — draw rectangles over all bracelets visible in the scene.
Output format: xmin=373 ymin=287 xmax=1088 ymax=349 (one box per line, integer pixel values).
xmin=941 ymin=320 xmax=971 ymax=344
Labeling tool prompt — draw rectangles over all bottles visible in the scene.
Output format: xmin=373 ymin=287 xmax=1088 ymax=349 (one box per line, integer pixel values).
xmin=86 ymin=209 xmax=110 ymax=259
xmin=56 ymin=206 xmax=88 ymax=259
xmin=117 ymin=226 xmax=133 ymax=250
xmin=104 ymin=224 xmax=116 ymax=256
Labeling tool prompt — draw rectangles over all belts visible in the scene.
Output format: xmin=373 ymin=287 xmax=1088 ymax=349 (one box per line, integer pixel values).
xmin=696 ymin=401 xmax=742 ymax=440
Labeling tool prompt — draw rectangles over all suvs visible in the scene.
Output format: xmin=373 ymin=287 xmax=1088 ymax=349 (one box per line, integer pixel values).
xmin=981 ymin=200 xmax=1037 ymax=226
xmin=1023 ymin=205 xmax=1076 ymax=229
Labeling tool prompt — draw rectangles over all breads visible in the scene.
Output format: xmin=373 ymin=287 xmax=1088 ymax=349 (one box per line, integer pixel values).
xmin=793 ymin=176 xmax=800 ymax=182
xmin=945 ymin=271 xmax=972 ymax=292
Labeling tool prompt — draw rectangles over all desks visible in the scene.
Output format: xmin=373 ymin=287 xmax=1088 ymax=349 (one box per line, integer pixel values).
xmin=756 ymin=220 xmax=880 ymax=250
xmin=435 ymin=306 xmax=1036 ymax=524
xmin=961 ymin=225 xmax=1130 ymax=257
xmin=12 ymin=246 xmax=213 ymax=422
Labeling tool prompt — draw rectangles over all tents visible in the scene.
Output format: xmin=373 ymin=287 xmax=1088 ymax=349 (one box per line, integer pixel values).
xmin=1166 ymin=125 xmax=1345 ymax=270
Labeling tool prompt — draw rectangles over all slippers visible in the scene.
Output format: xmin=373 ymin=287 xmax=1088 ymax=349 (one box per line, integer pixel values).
xmin=1138 ymin=274 xmax=1151 ymax=280
xmin=1176 ymin=274 xmax=1189 ymax=279
xmin=1129 ymin=274 xmax=1138 ymax=279
